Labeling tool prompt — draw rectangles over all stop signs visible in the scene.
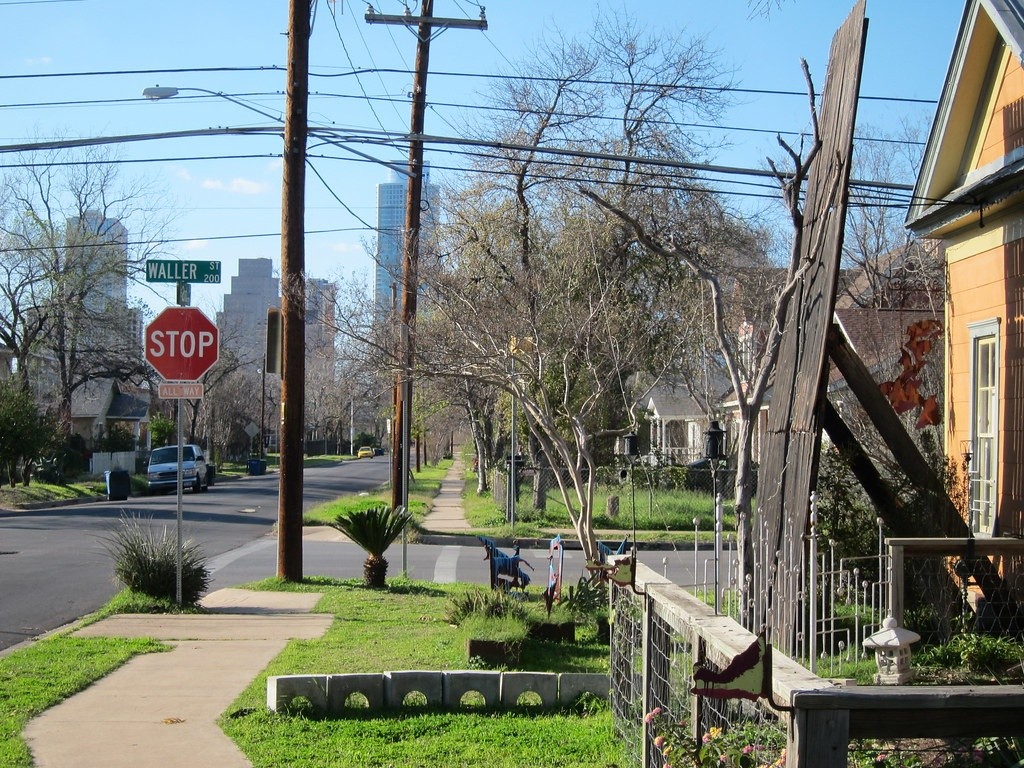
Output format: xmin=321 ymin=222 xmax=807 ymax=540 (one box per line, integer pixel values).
xmin=144 ymin=306 xmax=220 ymax=381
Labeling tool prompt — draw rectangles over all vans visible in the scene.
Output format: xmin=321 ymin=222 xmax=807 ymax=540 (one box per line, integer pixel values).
xmin=149 ymin=444 xmax=207 ymax=492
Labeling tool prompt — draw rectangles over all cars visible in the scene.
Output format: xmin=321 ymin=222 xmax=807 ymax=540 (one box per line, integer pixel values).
xmin=358 ymin=447 xmax=373 ymax=459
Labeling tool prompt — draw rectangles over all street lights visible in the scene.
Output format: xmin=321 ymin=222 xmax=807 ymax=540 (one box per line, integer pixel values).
xmin=143 ymin=86 xmax=424 ymax=514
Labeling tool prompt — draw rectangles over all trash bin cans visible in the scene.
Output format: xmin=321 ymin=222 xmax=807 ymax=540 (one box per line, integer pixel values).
xmin=104 ymin=470 xmax=128 ymax=500
xmin=248 ymin=460 xmax=261 ymax=475
xmin=206 ymin=465 xmax=216 ymax=485
xmin=260 ymin=461 xmax=266 ymax=475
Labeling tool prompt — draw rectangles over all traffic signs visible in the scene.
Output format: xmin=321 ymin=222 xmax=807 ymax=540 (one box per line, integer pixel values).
xmin=146 ymin=258 xmax=221 ymax=283
xmin=157 ymin=384 xmax=204 ymax=399
